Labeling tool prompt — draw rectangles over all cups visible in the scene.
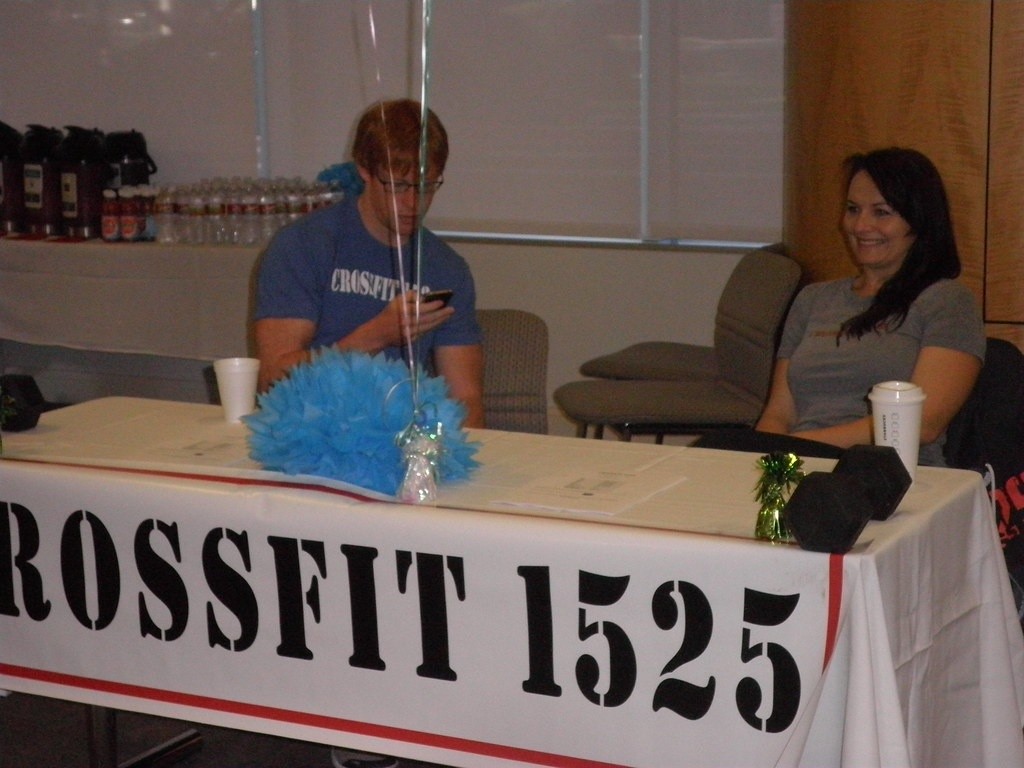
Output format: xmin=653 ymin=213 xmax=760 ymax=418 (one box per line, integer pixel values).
xmin=213 ymin=358 xmax=260 ymax=422
xmin=868 ymin=382 xmax=928 ymax=490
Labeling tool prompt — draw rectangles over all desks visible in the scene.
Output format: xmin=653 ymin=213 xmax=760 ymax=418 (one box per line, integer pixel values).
xmin=0 ymin=390 xmax=1024 ymax=768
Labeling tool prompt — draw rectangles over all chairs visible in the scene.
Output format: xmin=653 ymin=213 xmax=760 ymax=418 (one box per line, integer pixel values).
xmin=471 ymin=309 xmax=550 ymax=436
xmin=577 ymin=240 xmax=787 ymax=446
xmin=552 ymin=248 xmax=806 ymax=441
xmin=947 ymin=336 xmax=1024 ymax=636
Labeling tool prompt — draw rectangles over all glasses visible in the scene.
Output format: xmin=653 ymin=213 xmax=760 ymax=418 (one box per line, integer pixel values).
xmin=375 ymin=172 xmax=444 ymax=194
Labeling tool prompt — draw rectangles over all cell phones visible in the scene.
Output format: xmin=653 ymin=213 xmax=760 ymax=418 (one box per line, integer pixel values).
xmin=423 ymin=290 xmax=452 ymax=309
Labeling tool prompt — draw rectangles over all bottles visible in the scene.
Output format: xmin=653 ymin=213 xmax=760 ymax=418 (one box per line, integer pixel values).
xmin=101 ymin=176 xmax=344 ymax=245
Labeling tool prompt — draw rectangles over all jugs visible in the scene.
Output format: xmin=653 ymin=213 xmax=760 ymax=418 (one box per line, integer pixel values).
xmin=0 ymin=122 xmax=156 ymax=238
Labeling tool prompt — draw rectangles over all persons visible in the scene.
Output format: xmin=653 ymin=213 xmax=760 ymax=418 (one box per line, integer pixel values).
xmin=252 ymin=98 xmax=487 ymax=429
xmin=688 ymin=146 xmax=986 ymax=467
